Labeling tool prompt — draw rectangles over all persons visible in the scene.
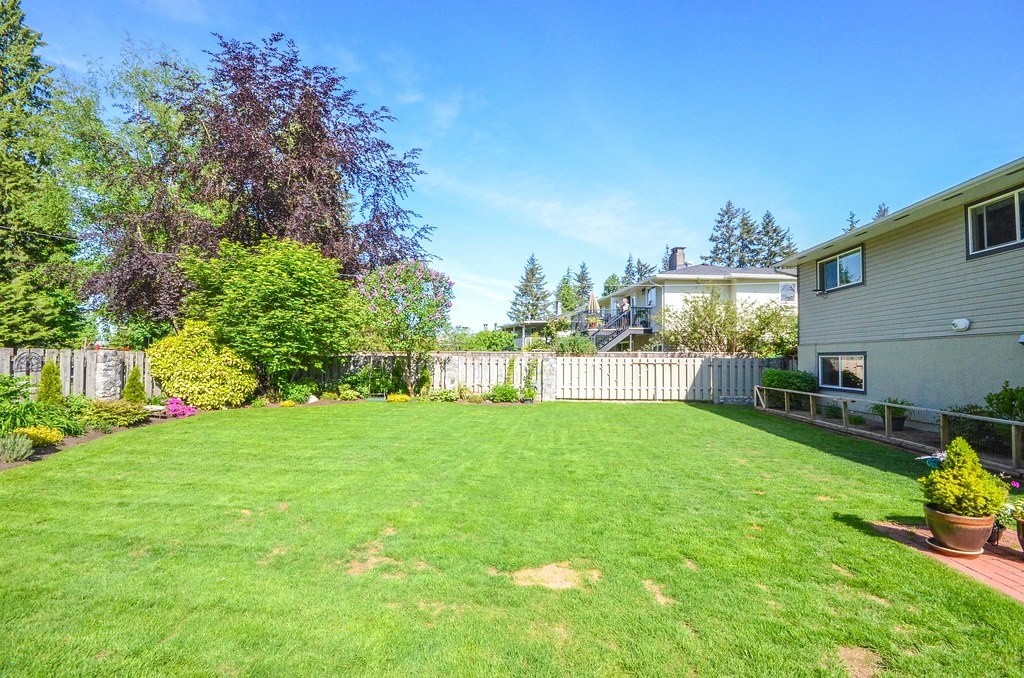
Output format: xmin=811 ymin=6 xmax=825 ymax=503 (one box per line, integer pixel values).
xmin=617 ymin=298 xmax=631 ymax=328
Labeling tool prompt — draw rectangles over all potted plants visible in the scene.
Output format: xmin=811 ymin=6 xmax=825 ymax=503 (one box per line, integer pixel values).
xmin=988 ymin=501 xmax=1012 ymax=543
xmin=866 ymin=396 xmax=922 ymax=430
xmin=1013 ymin=496 xmax=1024 ymax=551
xmin=917 ymin=437 xmax=1009 ymax=561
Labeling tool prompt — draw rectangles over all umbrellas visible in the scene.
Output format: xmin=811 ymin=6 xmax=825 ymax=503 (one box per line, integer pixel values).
xmin=587 ymin=290 xmax=600 ymax=328
